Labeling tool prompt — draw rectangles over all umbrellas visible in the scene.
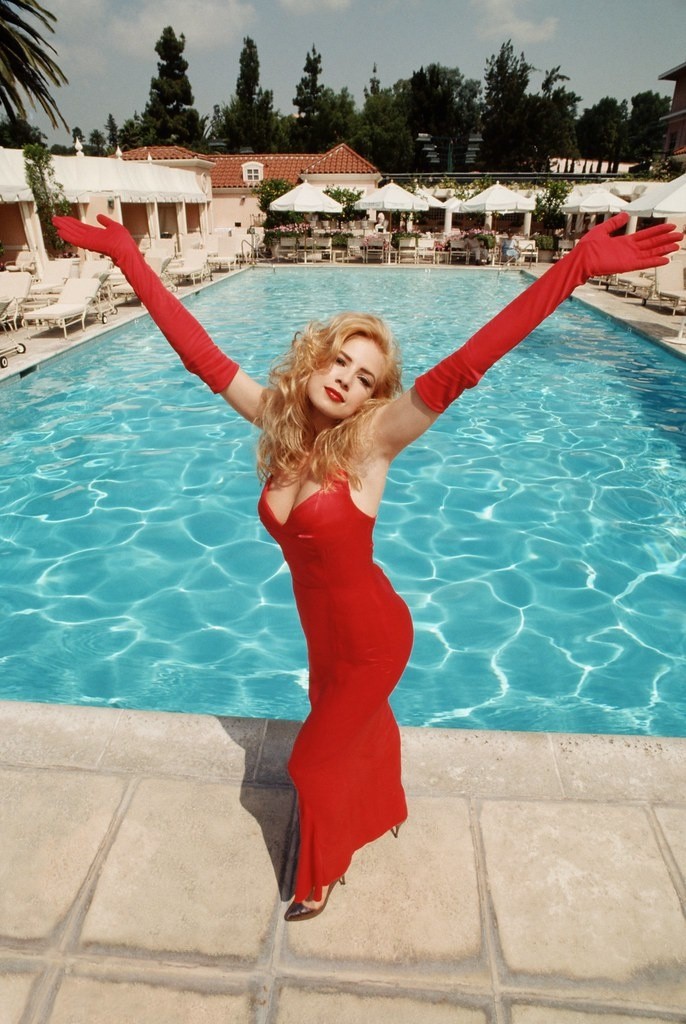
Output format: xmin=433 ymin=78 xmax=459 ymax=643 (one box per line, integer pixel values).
xmin=270 ymin=179 xmax=342 ymax=263
xmin=355 ymin=180 xmax=535 ymax=265
xmin=622 ymin=172 xmax=686 ymax=218
xmin=560 ymin=189 xmax=629 ymax=285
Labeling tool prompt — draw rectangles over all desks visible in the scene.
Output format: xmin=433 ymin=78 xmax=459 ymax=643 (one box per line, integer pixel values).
xmin=384 ymin=251 xmax=399 ymax=263
xmin=331 ymin=250 xmax=347 ymax=264
xmin=436 ymin=252 xmax=450 ymax=263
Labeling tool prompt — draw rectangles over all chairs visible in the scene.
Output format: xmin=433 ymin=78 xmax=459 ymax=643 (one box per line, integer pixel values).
xmin=0 ymin=226 xmax=686 ymax=368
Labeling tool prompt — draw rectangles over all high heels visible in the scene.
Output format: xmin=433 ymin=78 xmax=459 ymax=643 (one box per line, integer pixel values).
xmin=284 ymin=876 xmax=345 ymax=922
xmin=394 ymin=818 xmax=405 ymax=837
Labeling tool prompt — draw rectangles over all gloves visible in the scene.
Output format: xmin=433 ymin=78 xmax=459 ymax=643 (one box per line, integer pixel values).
xmin=51 ymin=214 xmax=239 ymax=394
xmin=415 ymin=211 xmax=684 ymax=412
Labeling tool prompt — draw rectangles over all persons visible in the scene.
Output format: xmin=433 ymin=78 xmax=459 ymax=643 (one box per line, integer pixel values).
xmin=0 ymin=240 xmax=6 ymax=268
xmin=374 ymin=213 xmax=388 ymax=250
xmin=465 ymin=232 xmax=481 ymax=266
xmin=63 ymin=244 xmax=79 ymax=258
xmin=52 ymin=213 xmax=684 ymax=922
xmin=500 ymin=232 xmax=519 ymax=267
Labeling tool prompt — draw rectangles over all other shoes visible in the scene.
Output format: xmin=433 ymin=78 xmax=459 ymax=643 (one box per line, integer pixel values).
xmin=476 ymin=261 xmax=480 ymax=265
xmin=487 ymin=260 xmax=490 ymax=264
